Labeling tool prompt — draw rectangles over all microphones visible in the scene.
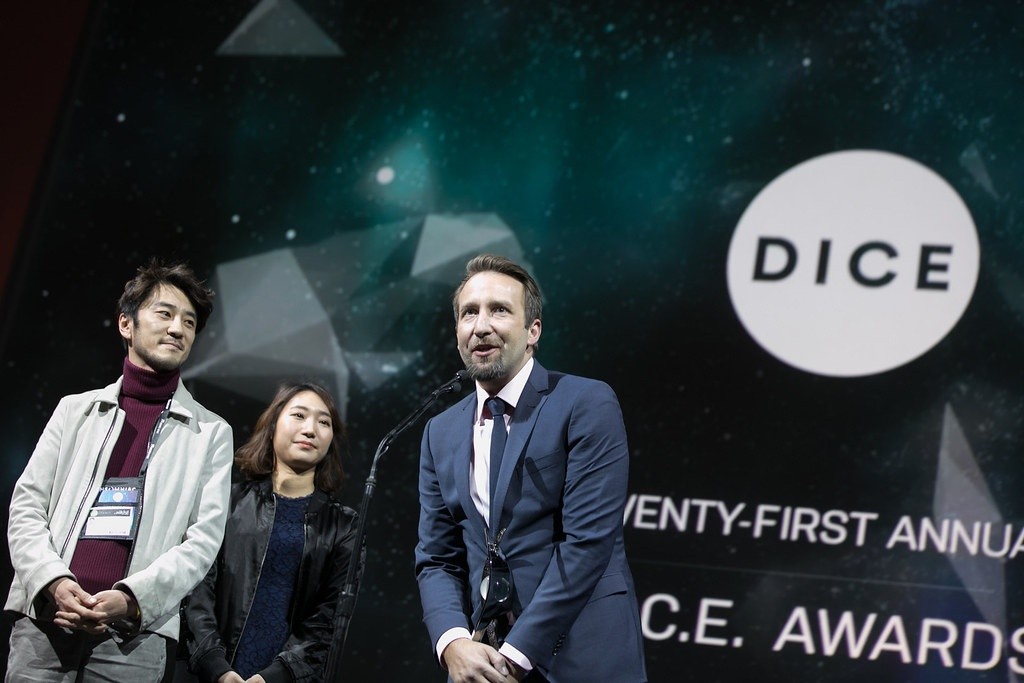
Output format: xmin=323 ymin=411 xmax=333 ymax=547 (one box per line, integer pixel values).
xmin=367 ymin=369 xmax=470 ymax=485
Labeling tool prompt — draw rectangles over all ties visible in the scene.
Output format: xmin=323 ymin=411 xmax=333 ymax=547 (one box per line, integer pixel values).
xmin=486 ymin=397 xmax=509 ymax=539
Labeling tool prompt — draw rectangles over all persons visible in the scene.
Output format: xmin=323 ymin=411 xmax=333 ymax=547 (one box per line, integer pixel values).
xmin=412 ymin=252 xmax=650 ymax=683
xmin=178 ymin=379 xmax=362 ymax=683
xmin=0 ymin=262 xmax=235 ymax=683
